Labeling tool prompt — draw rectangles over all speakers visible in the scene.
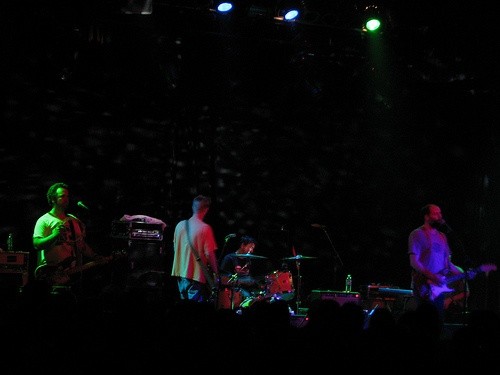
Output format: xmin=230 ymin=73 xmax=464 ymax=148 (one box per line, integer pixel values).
xmin=312 ymin=289 xmax=361 ymax=307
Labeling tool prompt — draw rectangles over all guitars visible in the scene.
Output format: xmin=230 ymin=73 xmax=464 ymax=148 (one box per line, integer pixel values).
xmin=33 ymin=255 xmax=114 ymax=286
xmin=425 ymin=264 xmax=497 ymax=302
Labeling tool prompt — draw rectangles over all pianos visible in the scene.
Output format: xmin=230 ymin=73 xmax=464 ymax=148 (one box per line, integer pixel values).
xmin=367 ymin=288 xmax=418 ymax=321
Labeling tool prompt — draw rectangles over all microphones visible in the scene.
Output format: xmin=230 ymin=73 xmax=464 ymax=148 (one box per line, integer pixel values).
xmin=440 ymin=220 xmax=451 ymax=231
xmin=311 ymin=224 xmax=325 ymax=228
xmin=228 ymin=234 xmax=237 ymax=237
xmin=77 ymin=201 xmax=89 ymax=210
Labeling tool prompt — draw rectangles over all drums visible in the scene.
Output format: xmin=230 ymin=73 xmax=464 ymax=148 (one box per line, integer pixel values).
xmin=258 ymin=269 xmax=296 ymax=305
xmin=290 ymin=315 xmax=312 ymax=328
xmin=309 ymin=290 xmax=361 ymax=308
xmin=214 ymin=275 xmax=256 ymax=311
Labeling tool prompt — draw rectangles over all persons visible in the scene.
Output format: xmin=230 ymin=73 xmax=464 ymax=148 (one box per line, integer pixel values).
xmin=170 ymin=195 xmax=219 ymax=302
xmin=408 ymin=204 xmax=463 ymax=301
xmin=32 ymin=183 xmax=105 ymax=271
xmin=220 ymin=234 xmax=257 ymax=290
xmin=0 ymin=303 xmax=500 ymax=375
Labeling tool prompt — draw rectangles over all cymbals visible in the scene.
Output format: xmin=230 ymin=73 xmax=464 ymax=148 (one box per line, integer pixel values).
xmin=227 ymin=253 xmax=268 ymax=260
xmin=281 ymin=254 xmax=318 ymax=261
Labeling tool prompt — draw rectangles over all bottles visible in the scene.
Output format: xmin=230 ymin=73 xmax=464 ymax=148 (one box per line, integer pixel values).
xmin=6 ymin=233 xmax=13 ymax=251
xmin=345 ymin=274 xmax=352 ymax=291
